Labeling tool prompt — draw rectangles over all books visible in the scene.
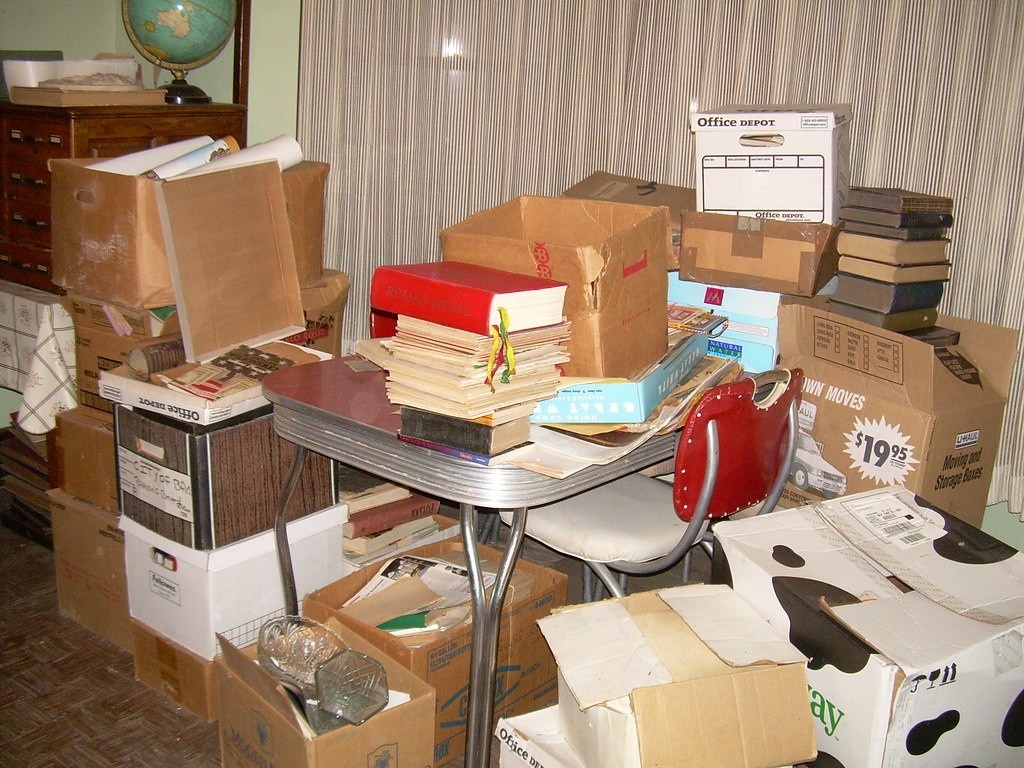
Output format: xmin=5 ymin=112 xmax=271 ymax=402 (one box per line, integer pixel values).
xmin=338 ymin=460 xmax=439 ymax=568
xmin=666 ymin=301 xmax=727 ymax=333
xmin=368 ymin=261 xmax=572 ymax=468
xmin=376 ymin=607 xmax=467 ymax=646
xmin=825 ymin=182 xmax=956 ymax=336
xmin=159 ymin=341 xmax=322 ymax=401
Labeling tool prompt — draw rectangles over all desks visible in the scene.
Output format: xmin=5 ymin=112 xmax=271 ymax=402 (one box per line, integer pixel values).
xmin=0 ymin=278 xmax=77 ymax=492
xmin=261 ymin=360 xmax=777 ymax=767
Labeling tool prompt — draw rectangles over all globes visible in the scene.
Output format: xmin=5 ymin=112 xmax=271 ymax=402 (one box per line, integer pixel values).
xmin=121 ymin=0 xmax=235 ymax=104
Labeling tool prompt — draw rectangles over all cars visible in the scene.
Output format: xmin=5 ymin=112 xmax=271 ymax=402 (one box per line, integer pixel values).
xmin=789 ymin=429 xmax=846 ymax=499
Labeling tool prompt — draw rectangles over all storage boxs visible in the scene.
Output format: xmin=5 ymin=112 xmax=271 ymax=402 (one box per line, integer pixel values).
xmin=45 ymin=99 xmax=1024 ymax=768
xmin=3 ymin=60 xmax=138 ymax=102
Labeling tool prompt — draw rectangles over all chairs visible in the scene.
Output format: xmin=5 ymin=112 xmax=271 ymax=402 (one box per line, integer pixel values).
xmin=500 ymin=368 xmax=806 ymax=598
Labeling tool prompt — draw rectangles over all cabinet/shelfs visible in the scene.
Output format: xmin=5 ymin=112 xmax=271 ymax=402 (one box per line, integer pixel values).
xmin=0 ymin=98 xmax=249 ymax=298
